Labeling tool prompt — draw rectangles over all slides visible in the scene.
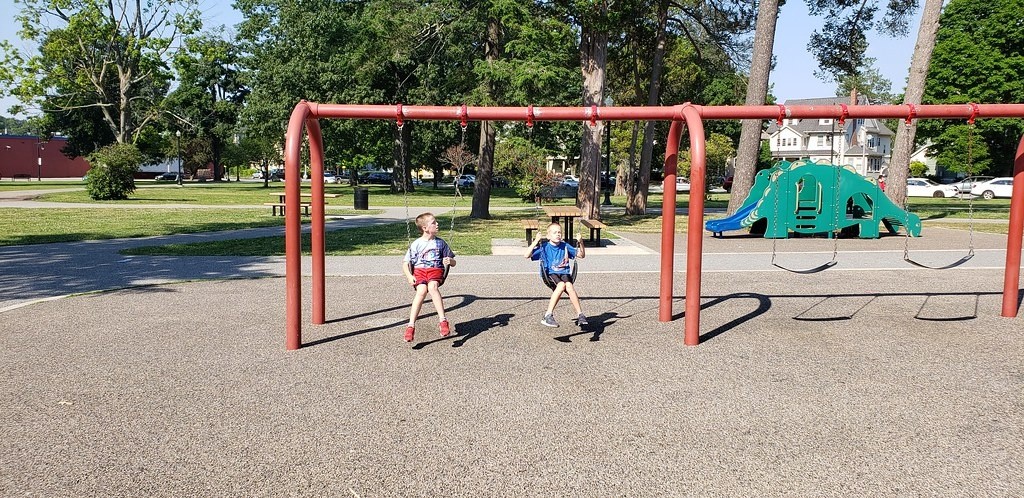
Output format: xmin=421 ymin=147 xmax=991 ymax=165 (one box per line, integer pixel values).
xmin=705 ymin=177 xmax=783 ymax=234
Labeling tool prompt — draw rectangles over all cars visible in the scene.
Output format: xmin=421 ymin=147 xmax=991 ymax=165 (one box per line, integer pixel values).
xmin=969 ymin=177 xmax=1014 ymax=199
xmin=537 ymin=173 xmax=616 ymax=198
xmin=723 ymin=176 xmax=734 ymax=194
xmin=155 ymin=172 xmax=186 ymax=181
xmin=334 ymin=171 xmax=422 ymax=185
xmin=660 ymin=177 xmax=693 ymax=191
xmin=324 ymin=172 xmax=336 ymax=184
xmin=949 ymin=175 xmax=995 ymax=192
xmin=490 ymin=176 xmax=509 ymax=187
xmin=268 ymin=168 xmax=285 ymax=180
xmin=452 ymin=174 xmax=475 ymax=189
xmin=252 ymin=170 xmax=266 ymax=179
xmin=905 ymin=178 xmax=960 ymax=198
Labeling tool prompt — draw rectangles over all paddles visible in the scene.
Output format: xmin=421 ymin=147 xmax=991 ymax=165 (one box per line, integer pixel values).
xmin=901 ymin=120 xmax=977 ymax=269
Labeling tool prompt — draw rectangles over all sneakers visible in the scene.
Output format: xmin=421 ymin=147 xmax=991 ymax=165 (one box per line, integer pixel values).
xmin=540 ymin=314 xmax=559 ymax=328
xmin=438 ymin=321 xmax=451 ymax=337
xmin=578 ymin=313 xmax=588 ymax=325
xmin=404 ymin=326 xmax=416 ymax=342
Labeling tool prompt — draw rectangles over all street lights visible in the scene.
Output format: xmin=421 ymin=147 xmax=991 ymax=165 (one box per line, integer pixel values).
xmin=602 ymin=94 xmax=614 ymax=205
xmin=176 ymin=130 xmax=183 ymax=185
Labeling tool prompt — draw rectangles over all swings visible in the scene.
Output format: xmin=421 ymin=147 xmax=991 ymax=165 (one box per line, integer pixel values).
xmin=768 ymin=121 xmax=845 ymax=276
xmin=525 ymin=117 xmax=597 ymax=290
xmin=400 ymin=119 xmax=469 ymax=289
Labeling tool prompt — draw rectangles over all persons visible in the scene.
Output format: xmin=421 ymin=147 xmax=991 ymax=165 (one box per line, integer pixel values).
xmin=403 ymin=213 xmax=456 ymax=342
xmin=878 ymin=175 xmax=884 ymax=192
xmin=523 ymin=222 xmax=589 ymax=328
